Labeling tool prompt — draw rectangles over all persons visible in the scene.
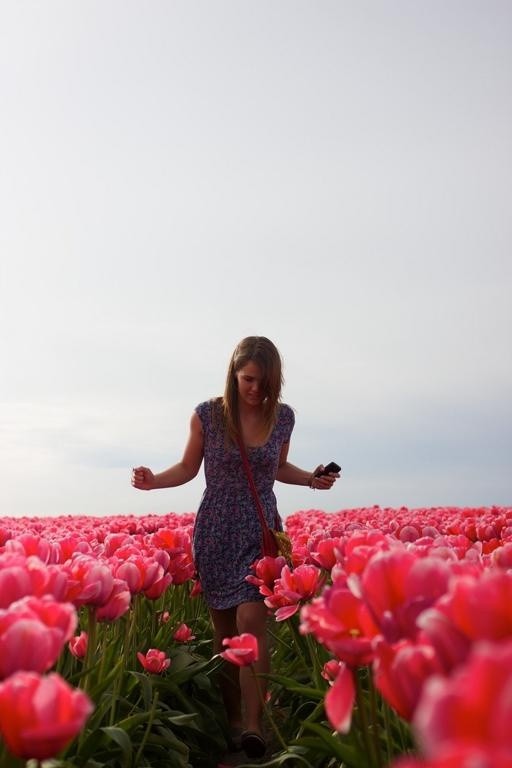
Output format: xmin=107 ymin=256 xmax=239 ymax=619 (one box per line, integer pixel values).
xmin=131 ymin=337 xmax=340 ymax=757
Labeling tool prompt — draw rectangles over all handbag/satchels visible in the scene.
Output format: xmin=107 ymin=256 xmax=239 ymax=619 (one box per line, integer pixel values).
xmin=262 ymin=516 xmax=294 ymax=569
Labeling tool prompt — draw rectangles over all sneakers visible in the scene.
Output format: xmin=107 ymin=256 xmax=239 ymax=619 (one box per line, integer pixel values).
xmin=228 ymin=726 xmax=246 ymax=753
xmin=241 ymin=730 xmax=268 ymax=760
